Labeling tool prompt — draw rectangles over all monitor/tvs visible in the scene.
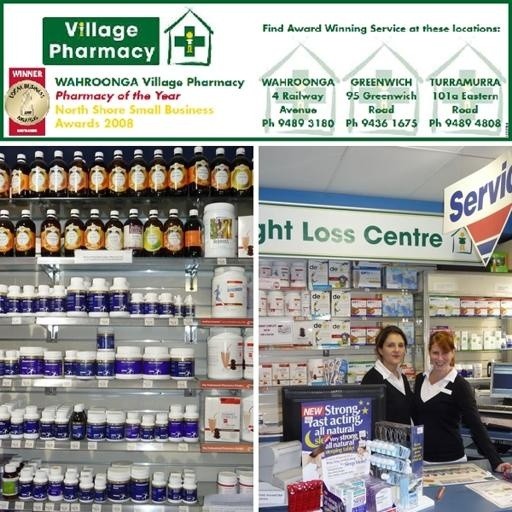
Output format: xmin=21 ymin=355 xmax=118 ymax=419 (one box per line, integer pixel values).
xmin=282 ymin=385 xmax=388 ymax=451
xmin=490 ymin=362 xmax=512 ymax=404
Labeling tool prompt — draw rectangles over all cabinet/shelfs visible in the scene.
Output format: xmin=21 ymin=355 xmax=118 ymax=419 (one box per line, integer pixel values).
xmin=258 ymin=265 xmax=512 ymax=440
xmin=0 ymin=183 xmax=254 ymax=512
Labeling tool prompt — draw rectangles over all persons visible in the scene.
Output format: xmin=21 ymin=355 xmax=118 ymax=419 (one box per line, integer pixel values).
xmin=358 ymin=324 xmax=414 ymax=444
xmin=414 ymin=329 xmax=512 ymax=479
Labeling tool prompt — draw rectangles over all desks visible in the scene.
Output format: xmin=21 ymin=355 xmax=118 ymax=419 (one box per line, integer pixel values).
xmin=259 ymin=455 xmax=512 ymax=512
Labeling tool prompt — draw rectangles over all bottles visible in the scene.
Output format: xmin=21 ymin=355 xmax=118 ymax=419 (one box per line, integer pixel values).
xmin=260 ymin=260 xmax=311 ymax=316
xmin=0 ymin=148 xmax=254 ymax=503
xmin=307 ymin=358 xmax=325 ymax=385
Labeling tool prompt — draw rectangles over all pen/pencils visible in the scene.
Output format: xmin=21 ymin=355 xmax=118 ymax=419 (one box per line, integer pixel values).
xmin=437 ymin=486 xmax=445 ymax=500
